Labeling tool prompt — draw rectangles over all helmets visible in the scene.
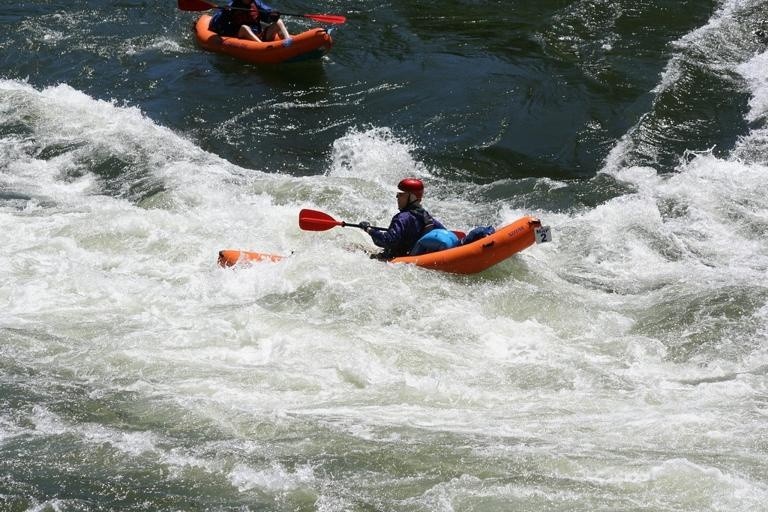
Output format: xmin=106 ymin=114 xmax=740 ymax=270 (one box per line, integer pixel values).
xmin=397 ymin=178 xmax=423 ymax=201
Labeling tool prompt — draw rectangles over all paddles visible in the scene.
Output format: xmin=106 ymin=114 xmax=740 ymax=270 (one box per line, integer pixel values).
xmin=299 ymin=208 xmax=465 ymax=242
xmin=177 ymin=1 xmax=348 ymax=25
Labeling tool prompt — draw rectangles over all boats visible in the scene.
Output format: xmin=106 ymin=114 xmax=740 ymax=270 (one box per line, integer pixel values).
xmin=219 ymin=214 xmax=541 ymax=277
xmin=193 ymin=13 xmax=332 ymax=62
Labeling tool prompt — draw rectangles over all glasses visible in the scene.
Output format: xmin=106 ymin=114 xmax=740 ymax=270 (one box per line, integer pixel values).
xmin=398 ymin=193 xmax=408 ymax=198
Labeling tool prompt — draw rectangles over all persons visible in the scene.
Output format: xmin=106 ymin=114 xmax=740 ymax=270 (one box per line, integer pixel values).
xmin=222 ymin=0 xmax=290 ymax=42
xmin=359 ymin=179 xmax=447 ymax=259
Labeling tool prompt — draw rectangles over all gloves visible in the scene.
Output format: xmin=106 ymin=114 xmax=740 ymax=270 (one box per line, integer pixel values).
xmin=359 ymin=222 xmax=370 ymax=233
